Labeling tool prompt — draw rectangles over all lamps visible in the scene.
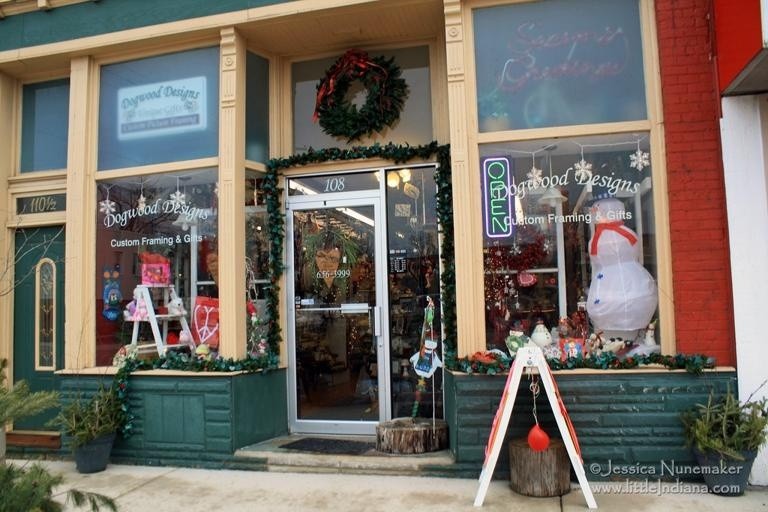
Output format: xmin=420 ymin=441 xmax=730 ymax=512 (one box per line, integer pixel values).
xmin=537 ymin=145 xmax=568 ymax=207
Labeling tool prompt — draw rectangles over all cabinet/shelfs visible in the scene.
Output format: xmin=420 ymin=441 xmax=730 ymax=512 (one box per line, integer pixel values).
xmin=484 ymin=182 xmax=569 ymax=320
xmin=188 ymin=204 xmax=272 ymax=330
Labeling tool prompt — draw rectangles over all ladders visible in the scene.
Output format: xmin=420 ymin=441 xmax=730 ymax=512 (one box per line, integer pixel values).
xmin=132 ymin=285 xmax=196 ymax=368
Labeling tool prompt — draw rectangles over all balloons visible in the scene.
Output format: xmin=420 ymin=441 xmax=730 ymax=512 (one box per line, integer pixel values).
xmin=526 ymin=423 xmax=550 ymax=451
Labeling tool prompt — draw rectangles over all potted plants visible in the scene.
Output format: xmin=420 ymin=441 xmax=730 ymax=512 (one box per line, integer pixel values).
xmin=677 ymin=379 xmax=767 ymax=496
xmin=44 ymin=377 xmax=123 ymax=474
